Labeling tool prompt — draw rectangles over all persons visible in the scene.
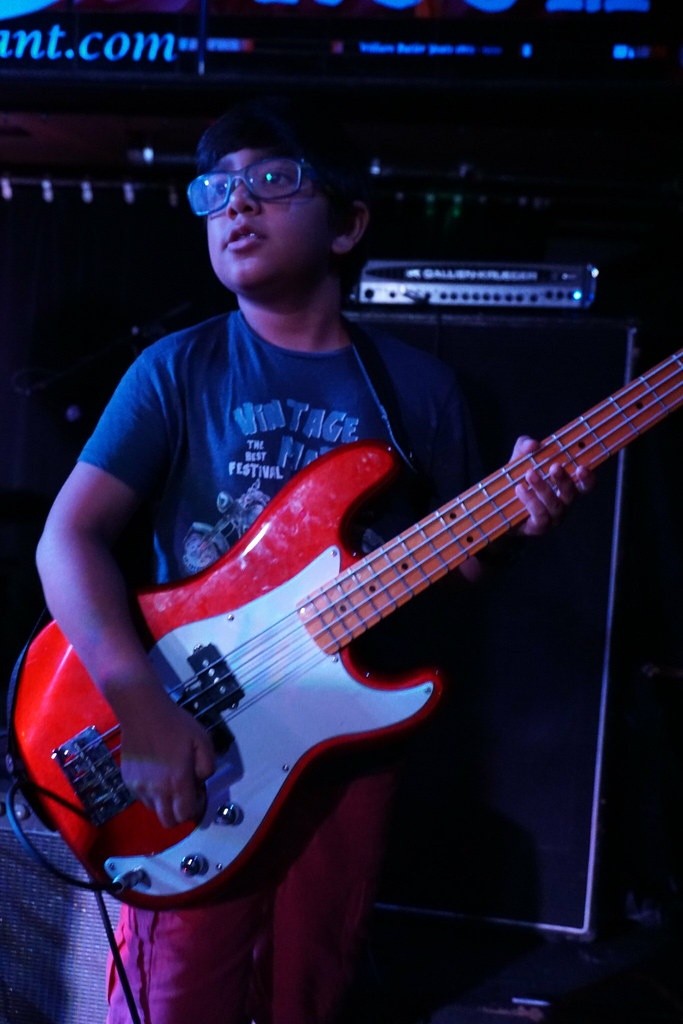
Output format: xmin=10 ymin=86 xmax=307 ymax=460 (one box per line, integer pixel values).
xmin=35 ymin=94 xmax=596 ymax=1024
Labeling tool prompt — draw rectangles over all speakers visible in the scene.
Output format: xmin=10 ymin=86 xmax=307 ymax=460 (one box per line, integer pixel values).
xmin=340 ymin=313 xmax=643 ymax=938
xmin=0 ymin=730 xmax=121 ymax=1024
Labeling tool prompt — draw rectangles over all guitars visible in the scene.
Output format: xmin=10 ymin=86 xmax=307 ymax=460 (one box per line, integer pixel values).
xmin=11 ymin=348 xmax=683 ymax=912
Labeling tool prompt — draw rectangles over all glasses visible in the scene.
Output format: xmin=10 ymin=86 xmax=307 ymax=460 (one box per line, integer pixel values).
xmin=186 ymin=155 xmax=313 ymax=216
